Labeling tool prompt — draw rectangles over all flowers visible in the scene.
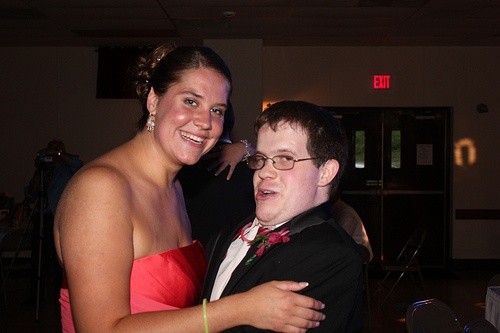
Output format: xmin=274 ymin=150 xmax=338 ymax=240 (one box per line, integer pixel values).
xmin=245 ymin=227 xmax=291 ymax=266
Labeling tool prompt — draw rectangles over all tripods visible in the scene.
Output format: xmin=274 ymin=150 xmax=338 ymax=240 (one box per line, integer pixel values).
xmin=1 ymin=169 xmax=54 ymax=321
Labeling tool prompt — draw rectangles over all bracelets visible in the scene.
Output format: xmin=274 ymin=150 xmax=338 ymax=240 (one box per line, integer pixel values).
xmin=202 ymin=297 xmax=211 ymax=333
xmin=240 ymin=136 xmax=250 ymax=162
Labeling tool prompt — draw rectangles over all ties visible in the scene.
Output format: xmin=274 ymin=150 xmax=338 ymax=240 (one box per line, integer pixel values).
xmin=210 ymin=225 xmax=261 ymax=301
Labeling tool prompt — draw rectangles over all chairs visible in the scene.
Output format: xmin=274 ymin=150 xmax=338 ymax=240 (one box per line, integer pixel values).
xmin=379 ymin=240 xmax=426 ymax=303
xmin=0 ymin=169 xmax=44 ymax=293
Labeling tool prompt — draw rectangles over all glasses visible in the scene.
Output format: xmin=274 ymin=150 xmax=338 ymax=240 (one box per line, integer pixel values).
xmin=246 ymin=154 xmax=325 ymax=171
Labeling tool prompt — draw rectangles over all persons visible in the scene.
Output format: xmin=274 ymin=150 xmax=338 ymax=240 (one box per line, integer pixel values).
xmin=15 ymin=89 xmax=253 ymax=333
xmin=203 ymin=98 xmax=365 ymax=333
xmin=52 ymin=40 xmax=328 ymax=333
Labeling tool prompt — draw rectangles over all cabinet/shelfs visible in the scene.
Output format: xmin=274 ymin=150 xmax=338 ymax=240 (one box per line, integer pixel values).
xmin=317 ymin=106 xmax=454 ymax=280
